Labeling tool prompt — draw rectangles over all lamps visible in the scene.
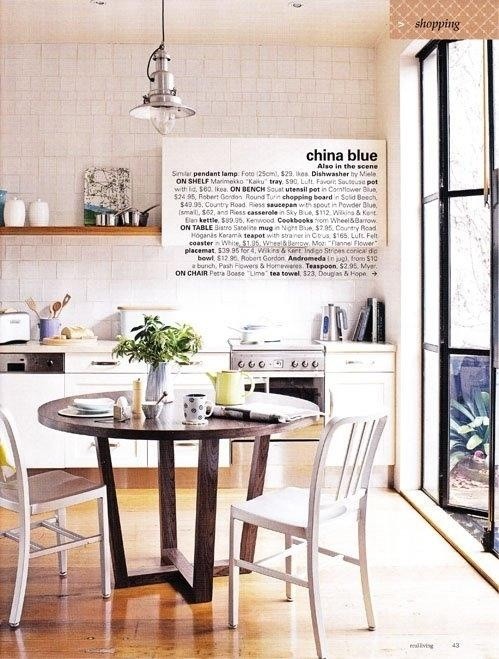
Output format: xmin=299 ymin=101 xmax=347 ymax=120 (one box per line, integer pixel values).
xmin=129 ymin=0 xmax=196 ymax=136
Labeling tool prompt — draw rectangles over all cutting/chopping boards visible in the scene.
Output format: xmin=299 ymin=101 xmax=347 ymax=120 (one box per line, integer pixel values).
xmin=42 ymin=337 xmax=98 ymax=346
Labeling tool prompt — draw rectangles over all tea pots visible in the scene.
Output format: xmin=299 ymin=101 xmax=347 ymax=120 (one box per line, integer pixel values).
xmin=205 ymin=370 xmax=255 ymax=406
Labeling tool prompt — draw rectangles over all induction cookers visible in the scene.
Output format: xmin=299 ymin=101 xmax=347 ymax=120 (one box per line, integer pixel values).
xmin=228 ymin=337 xmax=326 ymax=371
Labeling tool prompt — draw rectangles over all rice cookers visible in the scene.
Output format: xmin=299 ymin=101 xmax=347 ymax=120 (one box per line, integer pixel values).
xmin=0 ymin=308 xmax=31 ymax=344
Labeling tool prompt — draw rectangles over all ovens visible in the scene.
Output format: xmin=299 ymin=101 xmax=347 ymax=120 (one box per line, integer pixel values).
xmin=242 ymin=371 xmax=325 ymax=413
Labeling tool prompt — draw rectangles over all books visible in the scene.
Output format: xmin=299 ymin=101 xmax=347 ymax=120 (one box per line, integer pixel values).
xmin=319 ymin=297 xmax=386 ymax=343
xmin=205 ymin=403 xmax=329 ymax=425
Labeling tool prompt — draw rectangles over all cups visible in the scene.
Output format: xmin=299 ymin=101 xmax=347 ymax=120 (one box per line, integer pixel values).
xmin=38 ymin=318 xmax=60 ymax=340
xmin=141 ymin=401 xmax=163 ymax=419
xmin=183 ymin=394 xmax=214 ymax=422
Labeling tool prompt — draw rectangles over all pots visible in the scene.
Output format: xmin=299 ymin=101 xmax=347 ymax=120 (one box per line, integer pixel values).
xmin=95 ymin=202 xmax=161 ymax=226
xmin=228 ymin=325 xmax=267 ymax=346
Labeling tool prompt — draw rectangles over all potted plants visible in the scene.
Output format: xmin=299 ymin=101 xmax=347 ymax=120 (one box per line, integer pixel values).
xmin=110 ymin=312 xmax=204 ymax=404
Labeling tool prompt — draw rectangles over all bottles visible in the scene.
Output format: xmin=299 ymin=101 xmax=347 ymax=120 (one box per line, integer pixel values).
xmin=3 ymin=196 xmax=49 ymax=226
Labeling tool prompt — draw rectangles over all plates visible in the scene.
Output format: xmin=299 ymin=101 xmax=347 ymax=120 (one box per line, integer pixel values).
xmin=68 ymin=398 xmax=115 ymax=413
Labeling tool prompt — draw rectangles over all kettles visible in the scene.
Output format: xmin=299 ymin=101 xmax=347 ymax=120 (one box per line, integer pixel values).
xmin=320 ymin=304 xmax=349 ymax=343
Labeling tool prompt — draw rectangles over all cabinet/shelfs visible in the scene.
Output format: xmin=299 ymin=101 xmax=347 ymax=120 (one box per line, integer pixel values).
xmin=325 ymin=352 xmax=395 ymax=487
xmin=1 ymin=353 xmax=230 ymax=489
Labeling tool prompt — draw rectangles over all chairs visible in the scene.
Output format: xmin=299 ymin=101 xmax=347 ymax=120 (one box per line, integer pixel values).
xmin=0 ymin=408 xmax=113 ymax=628
xmin=230 ymin=410 xmax=388 ymax=658
